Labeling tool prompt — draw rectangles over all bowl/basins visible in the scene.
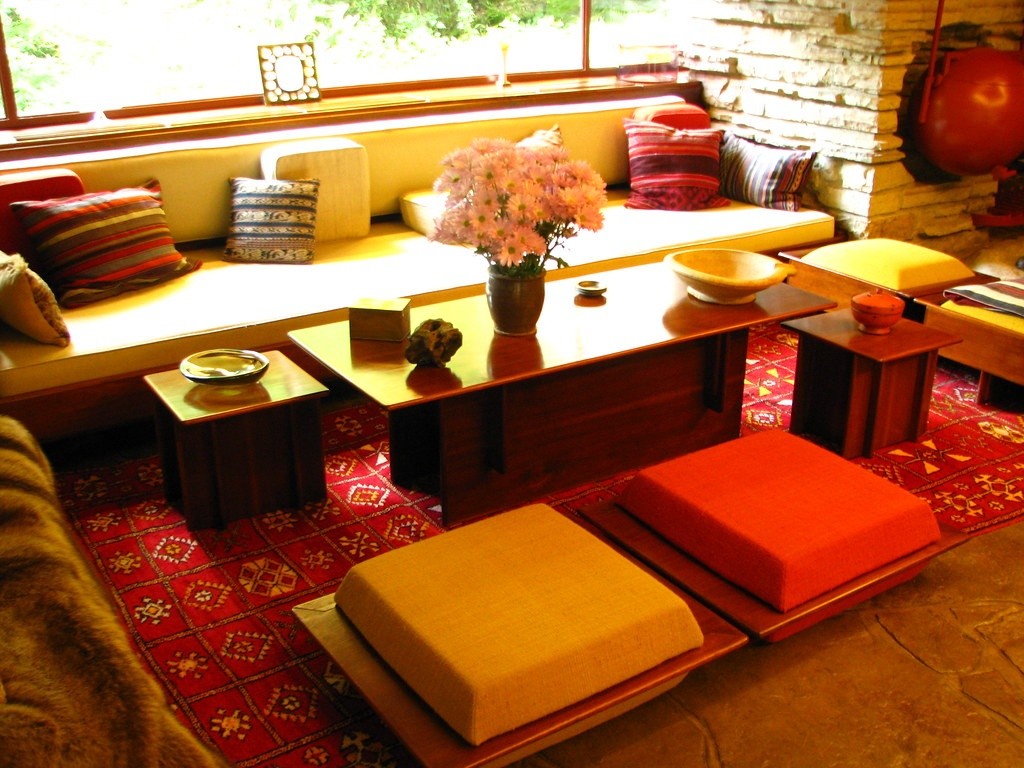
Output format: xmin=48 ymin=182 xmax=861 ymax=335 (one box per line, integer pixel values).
xmin=663 ymin=249 xmax=797 ymax=305
xmin=850 ymin=287 xmax=905 ymax=334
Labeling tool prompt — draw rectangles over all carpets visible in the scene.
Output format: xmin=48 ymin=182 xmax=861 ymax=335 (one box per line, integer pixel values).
xmin=37 ymin=310 xmax=1024 ymax=768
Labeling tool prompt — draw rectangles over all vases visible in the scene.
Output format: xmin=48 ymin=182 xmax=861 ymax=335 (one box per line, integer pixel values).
xmin=487 ymin=258 xmax=543 ymax=335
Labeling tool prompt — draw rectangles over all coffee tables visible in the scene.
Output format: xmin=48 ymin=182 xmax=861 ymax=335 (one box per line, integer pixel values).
xmin=289 ymin=259 xmax=836 ymax=494
xmin=140 ymin=352 xmax=329 ymax=533
xmin=778 ymin=303 xmax=963 ymax=463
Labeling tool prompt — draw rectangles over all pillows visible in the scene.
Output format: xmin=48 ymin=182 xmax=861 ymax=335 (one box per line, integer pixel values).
xmin=260 ymin=139 xmax=372 ymax=239
xmin=0 ymin=252 xmax=71 ymax=348
xmin=618 ymin=117 xmax=732 ymax=212
xmin=221 ymin=177 xmax=321 ymax=266
xmin=0 ymin=169 xmax=88 ymax=255
xmin=710 ymin=133 xmax=823 ymax=213
xmin=631 ymin=104 xmax=709 ymax=136
xmin=9 ymin=178 xmax=203 ymax=305
xmin=397 ymin=186 xmax=479 ymax=241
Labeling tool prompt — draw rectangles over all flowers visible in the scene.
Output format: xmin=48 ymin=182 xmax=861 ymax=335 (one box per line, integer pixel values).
xmin=437 ymin=137 xmax=609 ymax=262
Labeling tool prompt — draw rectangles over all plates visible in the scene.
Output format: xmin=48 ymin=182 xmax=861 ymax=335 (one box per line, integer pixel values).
xmin=180 ymin=349 xmax=270 ymax=386
xmin=578 ymin=281 xmax=608 ymax=296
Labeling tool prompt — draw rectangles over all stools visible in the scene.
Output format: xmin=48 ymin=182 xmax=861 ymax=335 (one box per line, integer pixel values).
xmin=776 ymin=235 xmax=1024 ymax=420
xmin=289 ymin=499 xmax=747 ymax=768
xmin=577 ymin=426 xmax=967 ymax=647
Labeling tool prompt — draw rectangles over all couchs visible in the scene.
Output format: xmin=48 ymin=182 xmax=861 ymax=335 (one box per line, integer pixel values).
xmin=0 ymin=83 xmax=840 ymax=447
xmin=0 ymin=418 xmax=214 ymax=768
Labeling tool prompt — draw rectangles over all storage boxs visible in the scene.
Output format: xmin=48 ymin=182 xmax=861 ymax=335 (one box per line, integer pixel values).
xmin=346 ymin=298 xmax=411 ymax=344
